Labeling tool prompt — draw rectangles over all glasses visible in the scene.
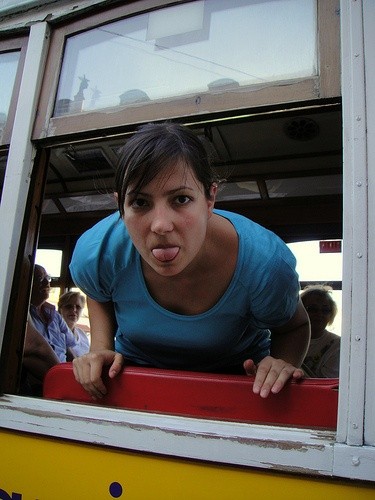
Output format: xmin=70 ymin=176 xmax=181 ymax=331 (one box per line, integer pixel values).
xmin=35 ymin=276 xmax=51 ymax=282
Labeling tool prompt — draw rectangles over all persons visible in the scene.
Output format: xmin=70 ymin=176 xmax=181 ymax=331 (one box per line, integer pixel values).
xmin=58 ymin=291 xmax=90 ymax=358
xmin=18 ymin=264 xmax=79 ymax=398
xmin=66 ymin=122 xmax=312 ymax=399
xmin=300 ymin=285 xmax=341 ymax=379
